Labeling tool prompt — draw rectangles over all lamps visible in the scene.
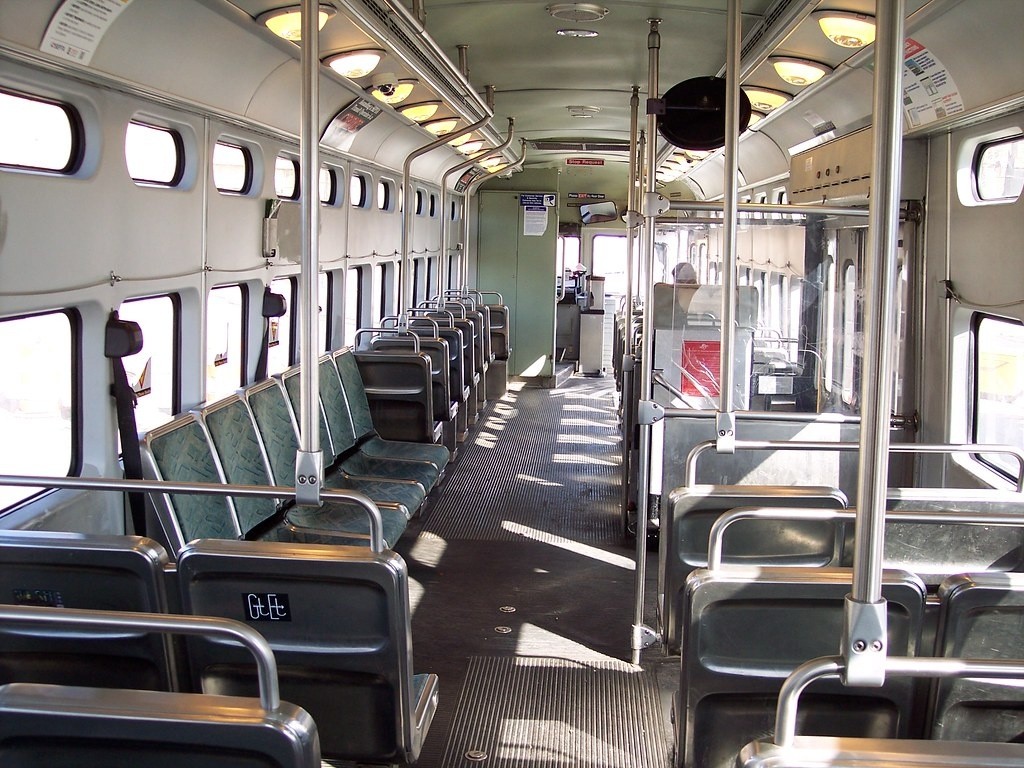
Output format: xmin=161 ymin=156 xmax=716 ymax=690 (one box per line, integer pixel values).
xmin=364 ymin=79 xmax=419 ymax=104
xmin=477 ymin=156 xmax=503 ymax=168
xmin=322 ymin=49 xmax=388 ymax=79
xmin=740 ymin=85 xmax=794 ymax=111
xmin=438 ymin=133 xmax=472 ymax=146
xmin=766 ymin=56 xmax=833 ymax=85
xmin=685 ymin=150 xmax=715 ymax=160
xmin=396 ymin=101 xmax=443 ymax=122
xmin=451 ymin=139 xmax=486 ymax=154
xmin=810 ymin=10 xmax=876 ymax=49
xmin=420 ymin=117 xmax=461 ymax=135
xmin=747 ymin=110 xmax=767 ymax=126
xmin=255 ymin=5 xmax=339 ymax=41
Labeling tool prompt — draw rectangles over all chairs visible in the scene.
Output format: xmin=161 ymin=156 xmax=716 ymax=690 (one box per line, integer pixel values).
xmin=330 ymin=346 xmax=452 ymax=477
xmin=137 ymin=412 xmax=239 ymax=562
xmin=352 ymin=290 xmax=513 ymax=487
xmin=234 ymin=376 xmax=409 ymax=550
xmin=318 ymin=354 xmax=440 ymax=496
xmin=188 ymin=392 xmax=386 ymax=550
xmin=272 ymin=362 xmax=426 ymax=519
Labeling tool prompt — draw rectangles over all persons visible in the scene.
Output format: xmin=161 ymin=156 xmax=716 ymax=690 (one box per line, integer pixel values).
xmin=672 ymin=263 xmax=697 ymax=284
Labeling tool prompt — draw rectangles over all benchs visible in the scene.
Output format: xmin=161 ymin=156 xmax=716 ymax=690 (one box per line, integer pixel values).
xmin=0 ymin=474 xmax=440 ymax=768
xmin=655 ymin=440 xmax=1024 ymax=657
xmin=0 ymin=604 xmax=332 ymax=768
xmin=612 ymin=293 xmax=804 ymax=452
xmin=668 ymin=505 xmax=1024 ymax=768
xmin=729 ymin=654 xmax=1024 ymax=768
